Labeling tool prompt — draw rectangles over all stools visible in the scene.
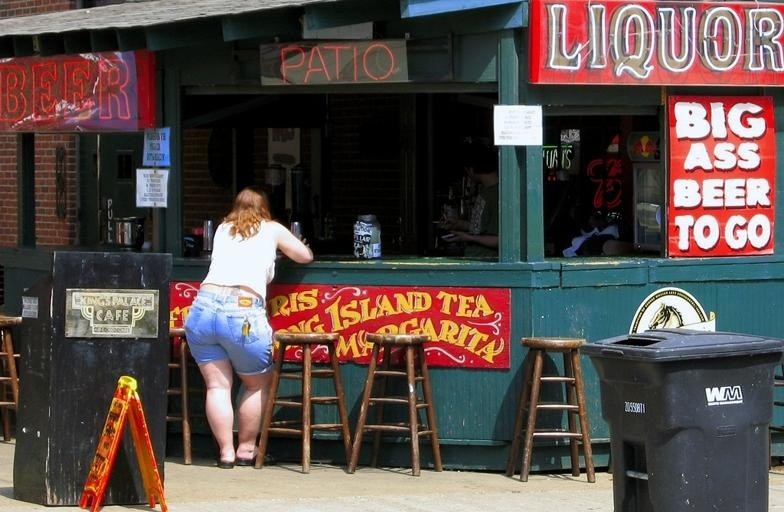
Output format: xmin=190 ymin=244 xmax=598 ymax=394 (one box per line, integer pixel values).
xmin=254 ymin=332 xmax=442 ymax=477
xmin=505 ymin=336 xmax=596 ymax=482
xmin=166 ymin=325 xmax=208 ymax=465
xmin=0 ymin=313 xmax=23 ymax=441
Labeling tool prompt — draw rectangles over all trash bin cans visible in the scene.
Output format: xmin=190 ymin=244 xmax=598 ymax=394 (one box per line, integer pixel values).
xmin=580 ymin=327 xmax=783 ymax=512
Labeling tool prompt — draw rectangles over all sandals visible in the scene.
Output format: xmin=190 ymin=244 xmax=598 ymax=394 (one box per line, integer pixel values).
xmin=220 ymin=454 xmax=274 ymax=468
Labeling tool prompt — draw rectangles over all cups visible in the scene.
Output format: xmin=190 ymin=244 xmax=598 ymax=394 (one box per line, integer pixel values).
xmin=291 ymin=221 xmax=300 ymax=239
xmin=203 ymin=220 xmax=213 ymax=252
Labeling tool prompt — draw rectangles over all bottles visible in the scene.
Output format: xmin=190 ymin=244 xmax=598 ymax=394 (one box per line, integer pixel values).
xmin=353 ymin=215 xmax=382 ymax=259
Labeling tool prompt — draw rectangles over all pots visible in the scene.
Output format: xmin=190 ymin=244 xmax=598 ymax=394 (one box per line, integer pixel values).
xmin=101 ymin=212 xmax=147 ymax=251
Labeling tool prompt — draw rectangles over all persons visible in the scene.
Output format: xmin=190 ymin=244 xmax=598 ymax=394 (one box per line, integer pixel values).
xmin=184 ymin=185 xmax=314 ymax=469
xmin=439 ymin=144 xmax=498 ymax=257
